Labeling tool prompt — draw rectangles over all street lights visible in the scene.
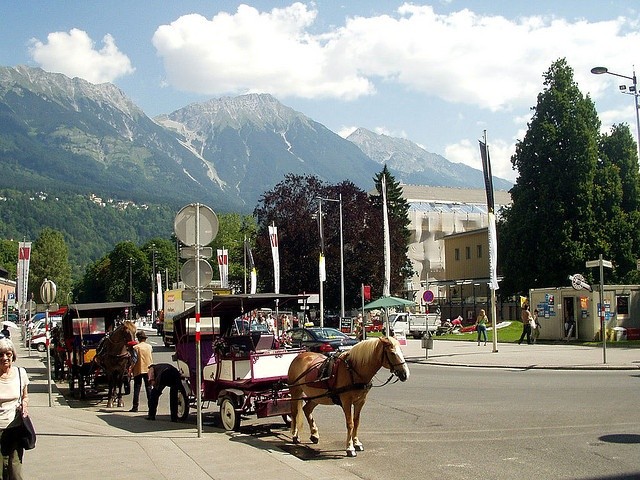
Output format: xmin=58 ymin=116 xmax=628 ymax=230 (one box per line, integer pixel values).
xmin=590 ymin=67 xmax=639 ymax=180
xmin=590 ymin=66 xmax=640 ymax=157
xmin=323 ymin=192 xmax=343 ymax=331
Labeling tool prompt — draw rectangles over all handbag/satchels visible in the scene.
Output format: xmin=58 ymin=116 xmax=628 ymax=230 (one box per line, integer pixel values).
xmin=7 ymin=366 xmax=36 ymax=450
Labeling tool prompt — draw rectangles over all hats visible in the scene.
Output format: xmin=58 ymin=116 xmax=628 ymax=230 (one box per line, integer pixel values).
xmin=137 ymin=331 xmax=148 ymax=338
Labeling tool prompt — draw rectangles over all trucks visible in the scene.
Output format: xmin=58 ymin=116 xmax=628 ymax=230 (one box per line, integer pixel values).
xmin=158 ymin=286 xmax=232 ymax=349
xmin=382 ymin=311 xmax=442 ymax=338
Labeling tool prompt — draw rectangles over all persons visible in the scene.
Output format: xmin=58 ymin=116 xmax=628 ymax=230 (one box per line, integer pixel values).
xmin=142 ymin=316 xmax=147 ymax=327
xmin=144 ymin=363 xmax=181 ymax=423
xmin=0 ymin=337 xmax=30 ymax=480
xmin=1 ymin=324 xmax=8 ymax=338
xmin=352 ymin=318 xmax=360 ymax=343
xmin=476 ymin=308 xmax=489 ymax=346
xmin=563 ymin=309 xmax=577 ymax=338
xmin=519 ymin=305 xmax=533 ymax=345
xmin=129 ymin=330 xmax=152 ymax=412
xmin=241 ymin=309 xmax=311 ymax=341
xmin=443 ymin=319 xmax=453 ymax=328
xmin=357 ymin=323 xmax=367 ymax=341
xmin=532 ymin=309 xmax=541 ymax=343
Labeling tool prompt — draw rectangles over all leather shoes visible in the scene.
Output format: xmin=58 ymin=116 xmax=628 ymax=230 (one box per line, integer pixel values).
xmin=145 ymin=413 xmax=156 ymax=420
xmin=129 ymin=405 xmax=138 ymax=412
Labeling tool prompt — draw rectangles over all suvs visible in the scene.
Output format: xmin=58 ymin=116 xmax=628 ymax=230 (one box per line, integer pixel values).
xmin=54 ymin=321 xmax=105 ymax=382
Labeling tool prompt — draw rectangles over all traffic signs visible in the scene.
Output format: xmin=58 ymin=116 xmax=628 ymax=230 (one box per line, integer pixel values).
xmin=586 ymin=261 xmax=600 ymax=269
xmin=423 ymin=290 xmax=435 ymax=302
xmin=601 ymin=260 xmax=612 ymax=270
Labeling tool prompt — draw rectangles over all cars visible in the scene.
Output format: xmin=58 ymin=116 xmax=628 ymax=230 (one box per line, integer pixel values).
xmin=277 ymin=327 xmax=358 ymax=358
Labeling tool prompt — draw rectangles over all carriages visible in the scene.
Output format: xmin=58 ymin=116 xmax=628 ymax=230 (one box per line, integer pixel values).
xmin=172 ymin=294 xmax=410 ymax=458
xmin=62 ymin=301 xmax=139 ymax=409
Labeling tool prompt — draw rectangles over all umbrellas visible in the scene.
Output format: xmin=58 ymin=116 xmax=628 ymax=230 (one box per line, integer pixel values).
xmin=1 ymin=319 xmax=18 ymax=331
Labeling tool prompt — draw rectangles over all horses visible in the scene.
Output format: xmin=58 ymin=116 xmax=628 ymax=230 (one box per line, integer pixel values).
xmin=97 ymin=320 xmax=138 ymax=407
xmin=288 ymin=335 xmax=410 ymax=457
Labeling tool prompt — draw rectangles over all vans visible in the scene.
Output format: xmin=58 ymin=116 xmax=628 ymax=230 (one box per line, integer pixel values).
xmin=29 ymin=317 xmax=64 ymax=352
